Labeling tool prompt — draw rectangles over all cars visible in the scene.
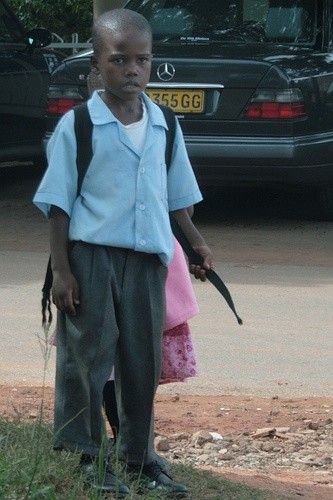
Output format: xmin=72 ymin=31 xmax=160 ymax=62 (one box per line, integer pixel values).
xmin=44 ymin=0 xmax=333 ymax=221
xmin=0 ymin=0 xmax=73 ymax=178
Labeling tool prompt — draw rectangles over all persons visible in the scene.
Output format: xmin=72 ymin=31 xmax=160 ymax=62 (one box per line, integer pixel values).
xmin=34 ymin=7 xmax=215 ymax=500
xmin=37 ymin=228 xmax=201 ymax=470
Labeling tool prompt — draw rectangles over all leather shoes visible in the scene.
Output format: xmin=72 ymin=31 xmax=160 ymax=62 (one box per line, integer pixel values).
xmin=124 ymin=459 xmax=188 ymax=495
xmin=72 ymin=460 xmax=130 ymax=496
